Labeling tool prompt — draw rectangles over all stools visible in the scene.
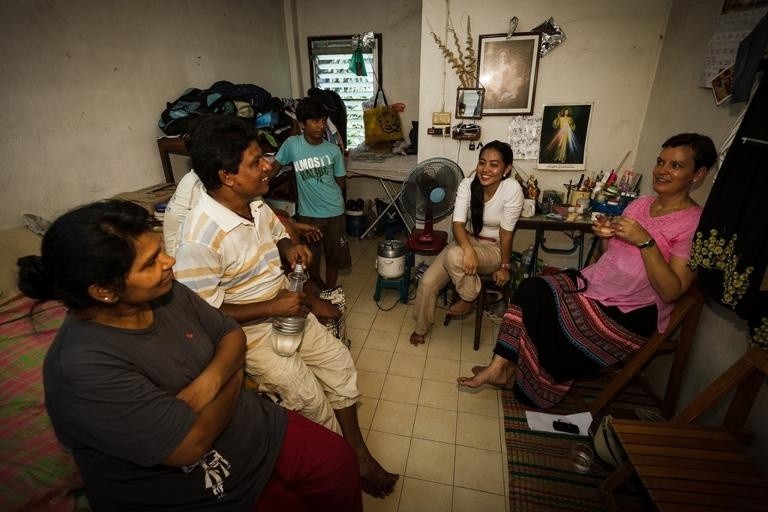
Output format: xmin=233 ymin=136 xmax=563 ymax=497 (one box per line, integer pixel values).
xmin=374 ymin=268 xmax=409 ymax=303
xmin=402 ymin=250 xmax=443 ymax=302
xmin=444 ymin=273 xmax=512 ymax=352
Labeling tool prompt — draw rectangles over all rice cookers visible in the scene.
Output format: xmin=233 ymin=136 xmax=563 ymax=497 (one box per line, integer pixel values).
xmin=375 ymin=240 xmax=406 ymax=280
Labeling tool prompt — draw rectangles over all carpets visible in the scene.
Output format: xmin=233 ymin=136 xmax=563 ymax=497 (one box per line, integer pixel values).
xmin=499 ymin=373 xmax=665 ymax=512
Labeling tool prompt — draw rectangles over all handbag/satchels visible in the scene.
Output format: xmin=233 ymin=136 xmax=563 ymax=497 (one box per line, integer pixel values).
xmin=363 ymin=88 xmax=403 ymax=146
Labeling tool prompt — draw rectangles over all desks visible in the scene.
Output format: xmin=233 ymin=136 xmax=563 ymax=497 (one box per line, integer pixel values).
xmin=345 ymin=148 xmax=418 ymax=242
xmin=515 ymin=205 xmax=602 ymax=277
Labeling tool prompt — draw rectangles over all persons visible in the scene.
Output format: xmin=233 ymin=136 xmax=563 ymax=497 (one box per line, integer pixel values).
xmin=267 ymin=98 xmax=347 ymax=292
xmin=554 ymin=108 xmax=576 ymax=161
xmin=456 ymin=133 xmax=717 ymax=411
xmin=17 ymin=198 xmax=364 ymax=510
xmin=171 ymin=113 xmax=399 ymax=500
xmin=485 ymin=49 xmax=519 ymax=102
xmin=409 ymin=141 xmax=526 ymax=348
xmin=162 ymin=170 xmax=341 ymax=322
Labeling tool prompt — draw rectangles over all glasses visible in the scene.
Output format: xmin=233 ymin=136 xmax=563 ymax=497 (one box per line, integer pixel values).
xmin=550 ymin=268 xmax=587 ymax=293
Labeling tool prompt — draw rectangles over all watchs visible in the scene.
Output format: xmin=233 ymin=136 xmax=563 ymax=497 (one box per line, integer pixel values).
xmin=635 ymin=237 xmax=657 ymax=249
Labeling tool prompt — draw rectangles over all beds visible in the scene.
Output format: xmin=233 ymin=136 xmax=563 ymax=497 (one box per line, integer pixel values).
xmin=2 ymin=208 xmax=89 ymax=511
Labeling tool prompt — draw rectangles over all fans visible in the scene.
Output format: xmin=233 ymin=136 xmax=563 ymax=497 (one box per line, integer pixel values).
xmin=401 ymin=156 xmax=465 ymax=253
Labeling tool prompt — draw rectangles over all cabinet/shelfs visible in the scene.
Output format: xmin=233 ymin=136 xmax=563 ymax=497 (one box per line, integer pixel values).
xmin=154 ymin=137 xmax=299 ymax=204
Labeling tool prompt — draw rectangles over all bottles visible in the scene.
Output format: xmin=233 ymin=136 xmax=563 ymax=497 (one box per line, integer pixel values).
xmin=575 ymin=196 xmax=590 ymax=213
xmin=517 ymin=244 xmax=534 ymax=281
xmin=268 ymin=264 xmax=306 ymax=356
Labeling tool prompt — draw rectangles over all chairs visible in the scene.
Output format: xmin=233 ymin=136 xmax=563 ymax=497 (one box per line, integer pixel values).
xmin=565 ymin=270 xmax=705 ymax=422
xmin=595 ymin=346 xmax=767 ymax=512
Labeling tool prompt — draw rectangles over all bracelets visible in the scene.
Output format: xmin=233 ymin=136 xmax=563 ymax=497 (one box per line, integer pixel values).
xmin=499 ymin=261 xmax=511 ymax=271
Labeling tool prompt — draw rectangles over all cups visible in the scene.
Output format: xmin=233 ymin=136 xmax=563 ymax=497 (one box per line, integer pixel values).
xmin=520 ymin=198 xmax=535 ymax=217
xmin=569 ymin=443 xmax=594 ymax=473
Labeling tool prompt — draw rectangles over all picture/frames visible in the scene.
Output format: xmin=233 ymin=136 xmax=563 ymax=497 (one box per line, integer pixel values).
xmin=475 ymin=31 xmax=541 ymax=116
xmin=455 ymin=87 xmax=484 ymax=119
xmin=536 ymin=101 xmax=595 ymax=171
